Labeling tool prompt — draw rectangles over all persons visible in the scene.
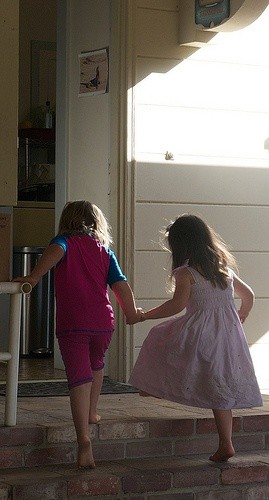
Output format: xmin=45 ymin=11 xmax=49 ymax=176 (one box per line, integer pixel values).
xmin=126 ymin=215 xmax=263 ymax=462
xmin=7 ymin=201 xmax=144 ymax=469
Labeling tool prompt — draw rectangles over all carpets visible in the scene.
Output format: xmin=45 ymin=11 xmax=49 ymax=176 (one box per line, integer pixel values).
xmin=0 ymin=376 xmax=141 ymax=396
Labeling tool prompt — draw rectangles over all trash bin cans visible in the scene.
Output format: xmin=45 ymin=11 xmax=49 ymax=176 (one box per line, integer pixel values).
xmin=13 ymin=245 xmax=54 ymax=359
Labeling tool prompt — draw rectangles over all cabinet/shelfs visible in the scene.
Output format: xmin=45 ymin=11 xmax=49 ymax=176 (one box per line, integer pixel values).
xmin=18 ymin=127 xmax=55 ymax=202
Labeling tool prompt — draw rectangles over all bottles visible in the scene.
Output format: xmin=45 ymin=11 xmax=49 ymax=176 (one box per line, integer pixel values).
xmin=42 ymin=101 xmax=53 ymax=128
